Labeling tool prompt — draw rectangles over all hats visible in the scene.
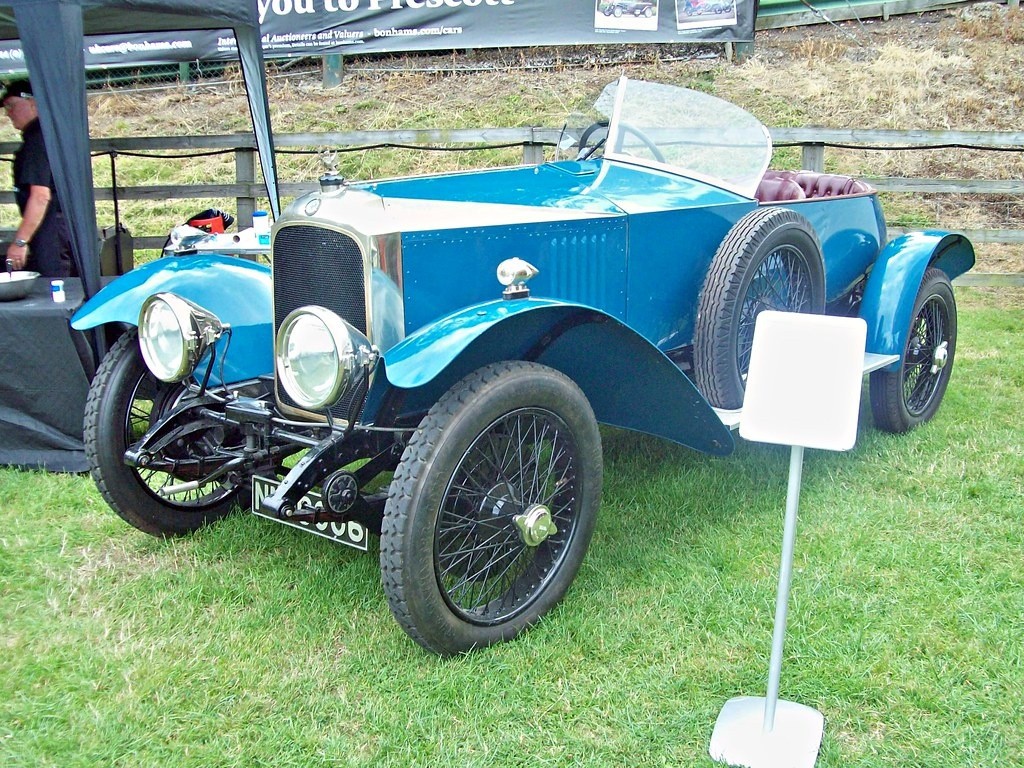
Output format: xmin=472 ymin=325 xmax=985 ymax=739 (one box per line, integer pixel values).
xmin=0 ymin=78 xmax=33 ymax=108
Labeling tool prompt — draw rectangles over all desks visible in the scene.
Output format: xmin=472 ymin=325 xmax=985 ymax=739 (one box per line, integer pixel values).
xmin=0 ymin=276 xmax=120 ymax=473
xmin=163 ymin=232 xmax=271 ymax=266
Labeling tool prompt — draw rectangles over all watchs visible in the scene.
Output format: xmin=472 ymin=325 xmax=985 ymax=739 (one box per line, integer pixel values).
xmin=13 ymin=239 xmax=27 ymax=247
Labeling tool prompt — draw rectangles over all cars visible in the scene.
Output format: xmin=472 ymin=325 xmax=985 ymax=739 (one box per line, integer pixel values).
xmin=81 ymin=77 xmax=981 ymax=662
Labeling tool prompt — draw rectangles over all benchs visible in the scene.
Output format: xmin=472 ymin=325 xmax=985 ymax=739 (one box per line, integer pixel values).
xmin=763 ymin=171 xmax=867 ymax=202
xmin=727 ymin=174 xmax=806 ymax=202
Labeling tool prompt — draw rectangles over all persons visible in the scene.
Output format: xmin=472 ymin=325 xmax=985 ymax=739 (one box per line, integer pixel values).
xmin=0 ymin=76 xmax=72 ymax=278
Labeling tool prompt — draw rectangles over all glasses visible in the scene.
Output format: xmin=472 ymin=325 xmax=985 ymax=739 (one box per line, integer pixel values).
xmin=5 ymin=97 xmax=29 ymax=110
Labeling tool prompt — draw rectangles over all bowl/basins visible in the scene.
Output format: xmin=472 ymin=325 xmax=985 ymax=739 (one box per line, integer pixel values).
xmin=0 ymin=271 xmax=39 ymax=301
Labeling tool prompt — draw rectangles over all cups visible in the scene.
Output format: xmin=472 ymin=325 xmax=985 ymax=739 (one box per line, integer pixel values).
xmin=253 ymin=211 xmax=268 ymax=233
xmin=50 ymin=281 xmax=65 ymax=302
